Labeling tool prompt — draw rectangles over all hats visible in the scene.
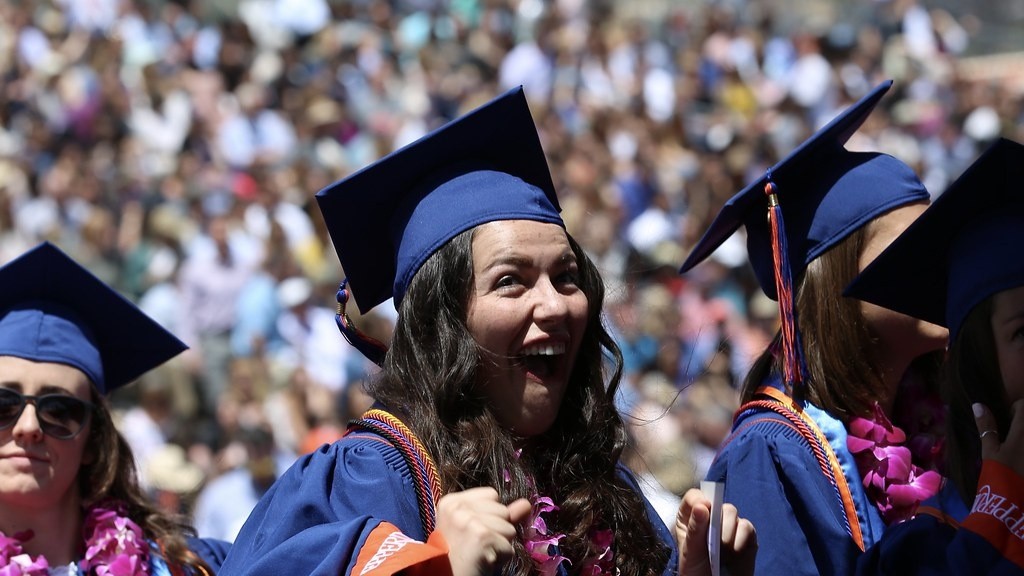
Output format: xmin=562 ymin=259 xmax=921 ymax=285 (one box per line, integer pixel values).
xmin=316 ymin=83 xmax=567 ymax=367
xmin=0 ymin=240 xmax=190 ymax=396
xmin=678 ymin=78 xmax=932 ymax=403
xmin=841 ymin=136 xmax=1024 ymax=341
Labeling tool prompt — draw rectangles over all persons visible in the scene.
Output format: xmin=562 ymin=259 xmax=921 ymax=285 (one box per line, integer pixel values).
xmin=0 ymin=241 xmax=233 ymax=576
xmin=680 ymin=78 xmax=1024 ymax=576
xmin=0 ymin=0 xmax=1024 ymax=544
xmin=218 ymin=85 xmax=760 ymax=576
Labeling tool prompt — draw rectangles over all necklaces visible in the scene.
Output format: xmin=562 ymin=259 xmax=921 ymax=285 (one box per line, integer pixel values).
xmin=0 ymin=508 xmax=149 ymax=576
xmin=847 ymin=368 xmax=953 ymax=525
xmin=504 ymin=448 xmax=614 ymax=576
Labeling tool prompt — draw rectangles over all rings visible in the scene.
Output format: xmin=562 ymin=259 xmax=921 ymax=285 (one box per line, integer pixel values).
xmin=980 ymin=430 xmax=997 ymax=437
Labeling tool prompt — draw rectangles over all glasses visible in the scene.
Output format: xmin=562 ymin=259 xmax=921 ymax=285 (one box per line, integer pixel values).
xmin=0 ymin=387 xmax=93 ymax=440
